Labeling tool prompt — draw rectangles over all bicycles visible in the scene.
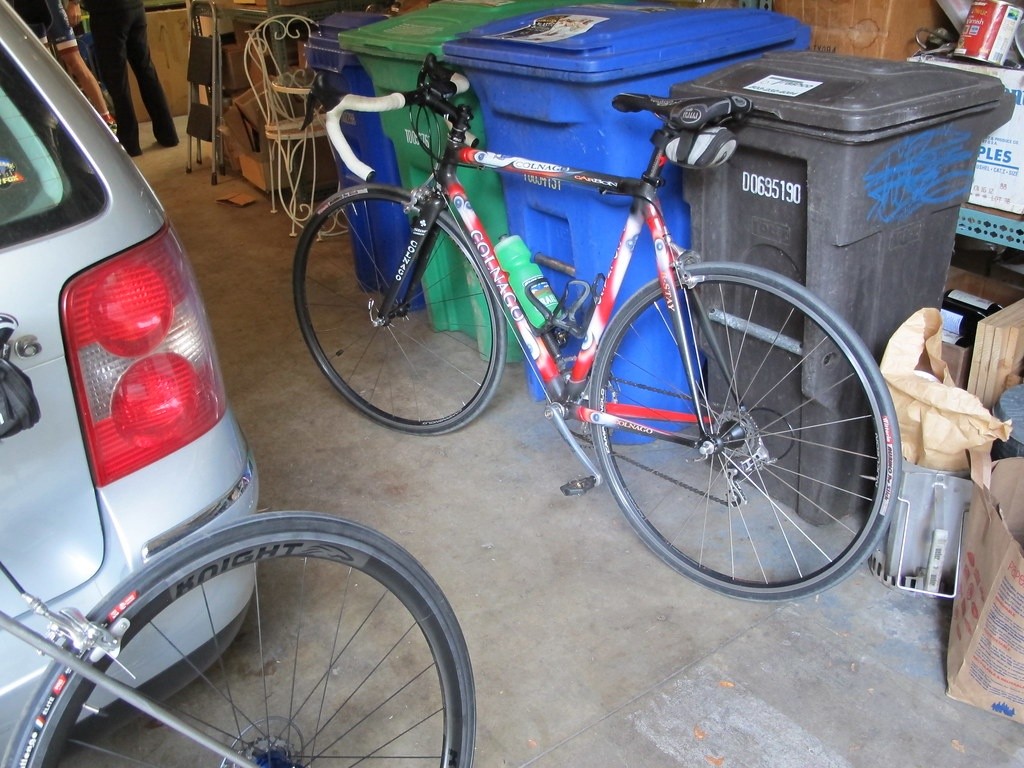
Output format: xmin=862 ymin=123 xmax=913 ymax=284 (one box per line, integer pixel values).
xmin=290 ymin=51 xmax=903 ymax=604
xmin=0 ymin=507 xmax=479 ymax=768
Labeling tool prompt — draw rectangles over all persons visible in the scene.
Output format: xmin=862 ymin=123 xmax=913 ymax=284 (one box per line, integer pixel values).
xmin=5 ymin=0 xmax=179 ymax=157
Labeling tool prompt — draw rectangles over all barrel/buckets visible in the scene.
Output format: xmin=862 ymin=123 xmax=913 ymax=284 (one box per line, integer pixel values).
xmin=953 ymin=0 xmax=1024 ymax=66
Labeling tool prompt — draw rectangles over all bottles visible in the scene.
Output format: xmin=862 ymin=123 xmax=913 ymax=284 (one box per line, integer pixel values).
xmin=492 ymin=232 xmax=563 ymax=329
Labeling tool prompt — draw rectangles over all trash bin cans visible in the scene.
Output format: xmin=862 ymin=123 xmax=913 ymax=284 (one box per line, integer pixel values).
xmin=441 ymin=3 xmax=813 ymax=447
xmin=668 ymin=49 xmax=1017 ymax=529
xmin=339 ymin=2 xmax=573 ymax=366
xmin=303 ymin=9 xmax=428 ymax=315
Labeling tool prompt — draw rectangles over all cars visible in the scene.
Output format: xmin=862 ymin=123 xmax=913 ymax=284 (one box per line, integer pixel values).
xmin=0 ymin=0 xmax=261 ymax=748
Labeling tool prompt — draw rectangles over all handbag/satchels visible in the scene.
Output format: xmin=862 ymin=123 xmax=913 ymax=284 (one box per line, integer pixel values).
xmin=944 ymin=455 xmax=1024 ymax=725
